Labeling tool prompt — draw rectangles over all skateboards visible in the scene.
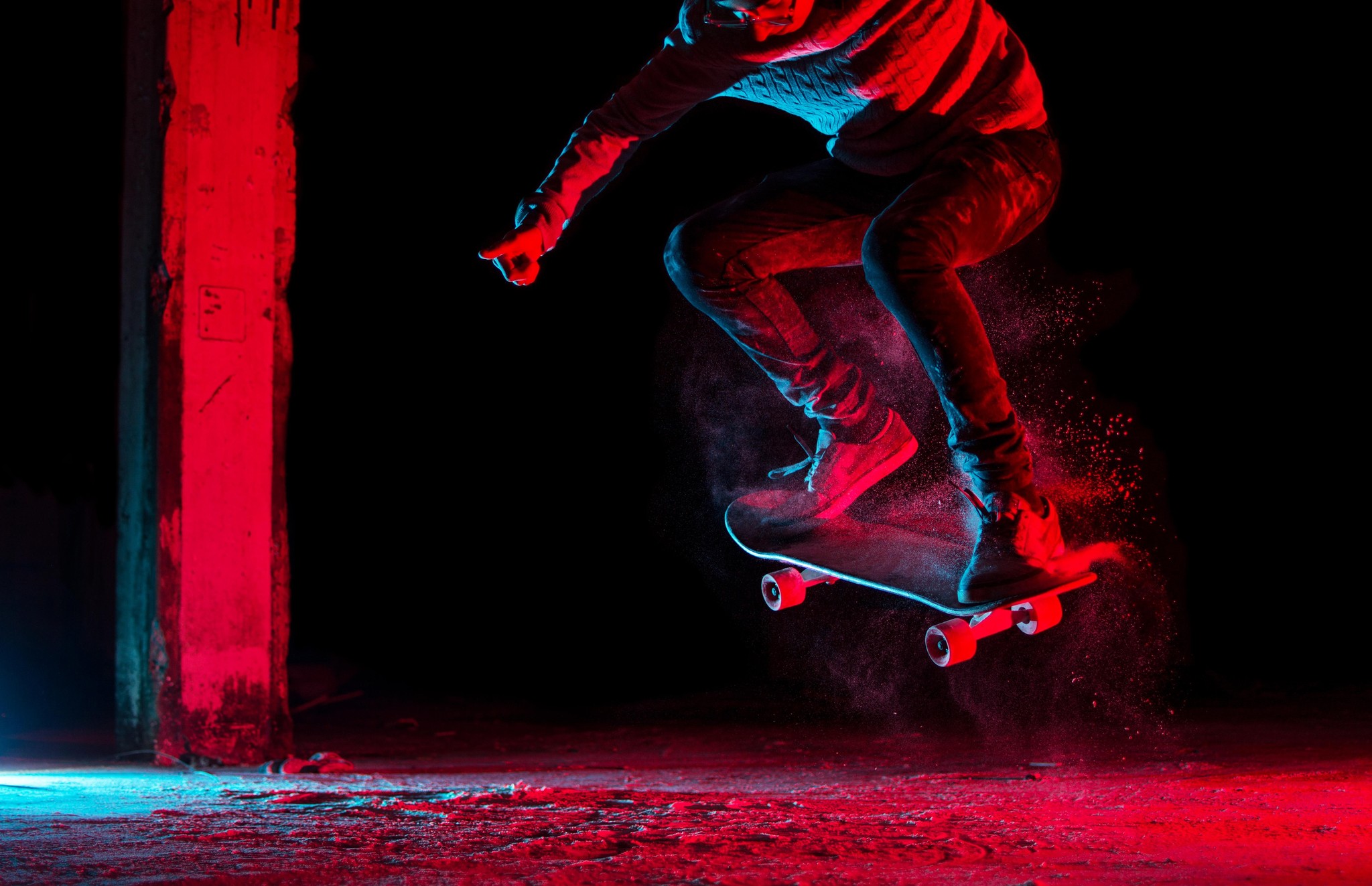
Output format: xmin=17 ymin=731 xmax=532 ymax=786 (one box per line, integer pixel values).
xmin=723 ymin=488 xmax=1099 ymax=669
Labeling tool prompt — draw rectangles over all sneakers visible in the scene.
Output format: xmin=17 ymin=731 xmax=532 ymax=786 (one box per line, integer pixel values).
xmin=758 ymin=409 xmax=919 ymax=540
xmin=949 ymin=481 xmax=1066 ymax=602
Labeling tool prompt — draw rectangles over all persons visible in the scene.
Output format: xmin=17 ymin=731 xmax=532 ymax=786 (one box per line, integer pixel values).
xmin=478 ymin=0 xmax=1065 ymax=603
